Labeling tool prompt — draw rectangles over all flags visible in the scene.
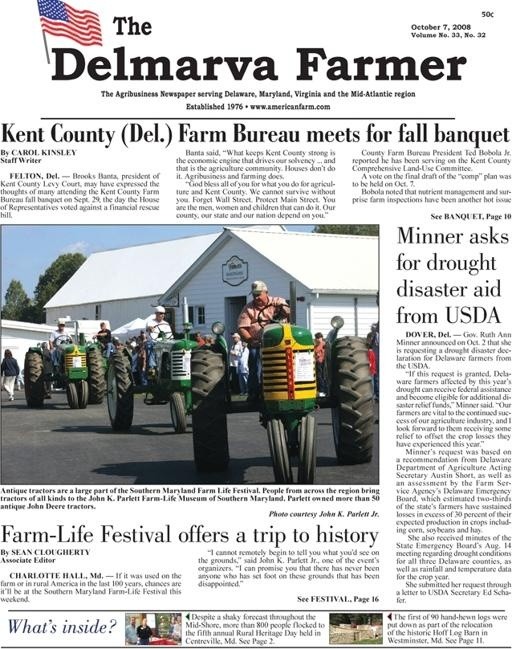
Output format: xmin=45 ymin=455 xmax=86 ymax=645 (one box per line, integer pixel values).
xmin=37 ymin=0 xmax=104 ymax=48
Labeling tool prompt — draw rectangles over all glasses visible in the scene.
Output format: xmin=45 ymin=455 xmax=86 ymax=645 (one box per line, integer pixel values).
xmin=157 ymin=312 xmax=165 ymax=315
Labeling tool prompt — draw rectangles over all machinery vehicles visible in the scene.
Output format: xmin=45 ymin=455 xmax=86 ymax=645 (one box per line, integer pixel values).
xmin=187 ymin=300 xmax=377 ymax=485
xmin=22 ymin=333 xmax=107 ymax=411
xmin=104 ymin=321 xmax=233 ymax=431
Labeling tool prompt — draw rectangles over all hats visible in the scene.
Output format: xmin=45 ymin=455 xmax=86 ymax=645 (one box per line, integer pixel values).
xmin=232 ymin=333 xmax=241 ymax=338
xmin=58 ymin=319 xmax=65 ymax=325
xmin=155 ymin=306 xmax=165 ymax=313
xmin=248 ymin=280 xmax=268 ymax=295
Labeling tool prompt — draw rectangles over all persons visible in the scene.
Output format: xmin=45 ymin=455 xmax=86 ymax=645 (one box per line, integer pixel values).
xmin=92 ymin=322 xmax=114 ymax=359
xmin=48 ymin=321 xmax=76 ymax=372
xmin=228 ymin=332 xmax=243 ymax=396
xmin=42 ymin=342 xmax=54 ymax=400
xmin=313 ymin=331 xmax=326 ymax=397
xmin=125 ymin=617 xmax=139 ymax=645
xmin=238 ymin=280 xmax=291 ymax=403
xmin=112 ymin=335 xmax=150 ymax=386
xmin=169 ymin=615 xmax=176 ymax=635
xmin=234 ymin=337 xmax=249 ymax=398
xmin=194 ymin=332 xmax=205 ymax=345
xmin=174 ymin=615 xmax=182 ymax=642
xmin=366 ymin=323 xmax=378 ymax=403
xmin=0 ymin=349 xmax=20 ymax=401
xmin=136 ymin=618 xmax=153 ymax=645
xmin=146 ymin=304 xmax=176 ymax=377
xmin=202 ymin=333 xmax=214 ymax=346
